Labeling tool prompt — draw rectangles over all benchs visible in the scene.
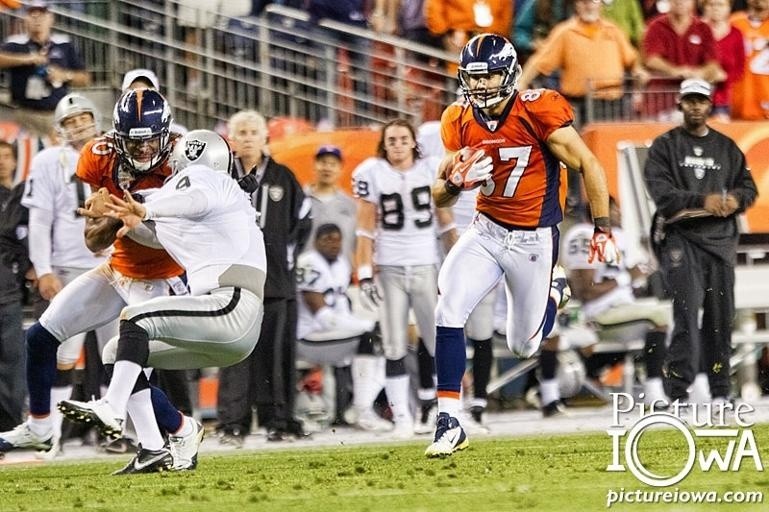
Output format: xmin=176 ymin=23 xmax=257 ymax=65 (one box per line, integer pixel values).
xmin=463 ymin=343 xmax=645 ymax=408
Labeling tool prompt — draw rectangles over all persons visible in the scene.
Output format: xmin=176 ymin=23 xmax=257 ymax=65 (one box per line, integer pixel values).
xmin=0 ymin=1 xmax=768 ymax=474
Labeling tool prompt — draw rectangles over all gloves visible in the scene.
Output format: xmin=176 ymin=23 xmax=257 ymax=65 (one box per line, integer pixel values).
xmin=358 ymin=277 xmax=384 ymax=313
xmin=588 ymin=231 xmax=622 ymax=271
xmin=445 ymin=145 xmax=494 ymax=196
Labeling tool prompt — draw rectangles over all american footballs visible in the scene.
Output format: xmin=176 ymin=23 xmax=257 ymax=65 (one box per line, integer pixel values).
xmin=445 ymin=147 xmax=489 ymax=190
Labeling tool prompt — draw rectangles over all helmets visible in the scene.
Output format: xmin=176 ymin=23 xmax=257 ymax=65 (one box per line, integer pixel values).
xmin=456 ymin=33 xmax=523 ymax=109
xmin=164 ymin=129 xmax=234 ymax=184
xmin=54 ymin=93 xmax=101 ymax=145
xmin=111 ymin=89 xmax=174 ymax=177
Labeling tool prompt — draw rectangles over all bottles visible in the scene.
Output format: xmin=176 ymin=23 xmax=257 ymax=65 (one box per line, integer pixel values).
xmin=33 ymin=51 xmax=62 ymax=89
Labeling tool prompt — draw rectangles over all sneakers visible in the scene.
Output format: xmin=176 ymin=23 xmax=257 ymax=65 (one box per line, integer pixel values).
xmin=344 ymin=405 xmax=394 ymax=432
xmin=551 ymin=264 xmax=571 ymax=303
xmin=0 ymin=422 xmax=55 ymax=453
xmin=424 ymin=412 xmax=469 ymax=458
xmin=167 ymin=416 xmax=206 ymax=472
xmin=413 ymin=396 xmax=438 ymax=434
xmin=56 ymin=394 xmax=127 ymax=443
xmin=110 ymin=443 xmax=174 ymax=476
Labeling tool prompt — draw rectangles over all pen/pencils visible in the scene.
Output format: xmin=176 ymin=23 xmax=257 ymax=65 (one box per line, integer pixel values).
xmin=721 ymin=186 xmax=728 ymax=205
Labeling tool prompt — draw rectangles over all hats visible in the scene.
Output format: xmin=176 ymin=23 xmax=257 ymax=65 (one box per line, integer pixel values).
xmin=679 ymin=78 xmax=711 ymax=100
xmin=122 ymin=68 xmax=160 ymax=93
xmin=316 ymin=146 xmax=342 ymax=161
